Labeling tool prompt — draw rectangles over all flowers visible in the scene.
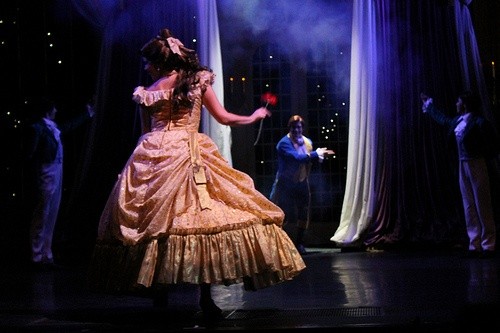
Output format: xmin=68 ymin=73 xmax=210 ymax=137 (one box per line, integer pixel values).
xmin=252 ymin=93 xmax=277 ymax=145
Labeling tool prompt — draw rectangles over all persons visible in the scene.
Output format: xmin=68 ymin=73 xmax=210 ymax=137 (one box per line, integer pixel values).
xmin=24 ymin=96 xmax=94 ymax=273
xmin=422 ymin=91 xmax=498 ymax=260
xmin=85 ymin=28 xmax=308 ymax=315
xmin=268 ymin=115 xmax=336 ymax=255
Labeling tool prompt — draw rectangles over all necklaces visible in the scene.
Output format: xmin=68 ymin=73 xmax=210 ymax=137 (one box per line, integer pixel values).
xmin=163 ymin=72 xmax=177 ymax=76
